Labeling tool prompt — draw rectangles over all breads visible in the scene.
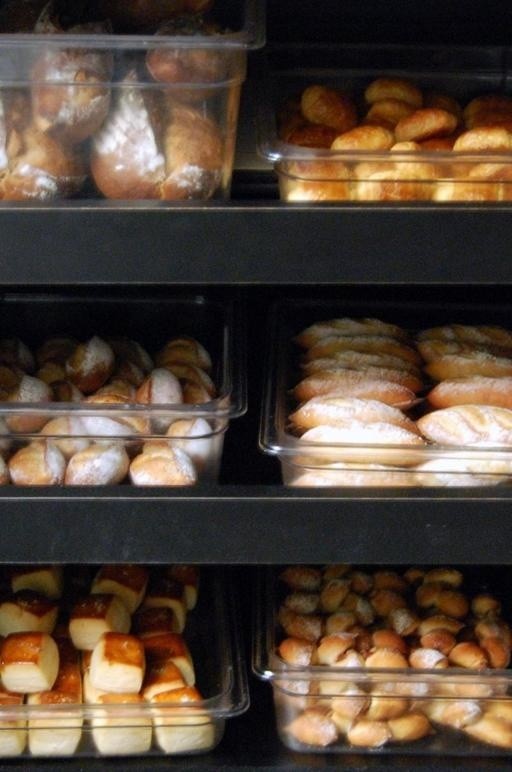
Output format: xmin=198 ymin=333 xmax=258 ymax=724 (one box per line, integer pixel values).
xmin=251 ymin=564 xmax=511 ymax=757
xmin=259 ymin=302 xmax=512 ymax=485
xmin=0 ymin=5 xmax=266 ymax=203
xmin=258 ymin=74 xmax=509 ymax=206
xmin=2 ymin=564 xmax=252 ymax=757
xmin=1 ymin=299 xmax=247 ymax=487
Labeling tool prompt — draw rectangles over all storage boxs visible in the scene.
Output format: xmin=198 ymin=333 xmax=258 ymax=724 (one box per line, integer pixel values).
xmin=254 ymin=111 xmax=511 ymax=204
xmin=258 ymin=302 xmax=512 ymax=486
xmin=0 ymin=294 xmax=252 ymax=487
xmin=0 ymin=576 xmax=250 ymax=753
xmin=0 ymin=1 xmax=265 ymax=207
xmin=250 ymin=577 xmax=512 ymax=754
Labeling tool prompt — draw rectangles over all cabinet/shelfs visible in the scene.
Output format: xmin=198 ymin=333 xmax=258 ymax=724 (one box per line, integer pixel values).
xmin=0 ymin=2 xmax=511 ymax=772
